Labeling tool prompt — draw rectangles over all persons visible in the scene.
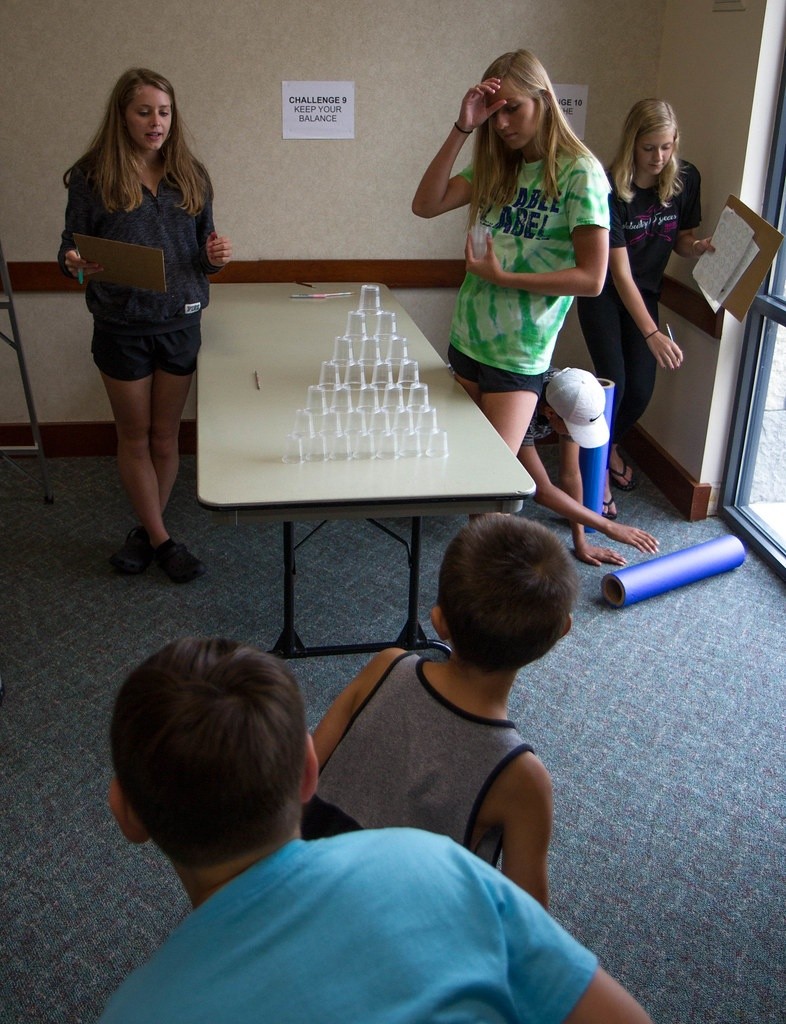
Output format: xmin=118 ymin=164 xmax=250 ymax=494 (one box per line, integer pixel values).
xmin=517 ymin=365 xmax=660 ymax=567
xmin=302 ymin=511 xmax=580 ymax=912
xmin=98 ymin=634 xmax=654 ymax=1024
xmin=412 ymin=47 xmax=612 ymax=523
xmin=57 ymin=68 xmax=234 ymax=583
xmin=577 ymin=97 xmax=717 ymax=522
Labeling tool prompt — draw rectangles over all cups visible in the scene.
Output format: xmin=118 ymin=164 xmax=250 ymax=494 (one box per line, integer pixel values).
xmin=470 ymin=226 xmax=492 ymax=260
xmin=281 ymin=285 xmax=448 ymax=463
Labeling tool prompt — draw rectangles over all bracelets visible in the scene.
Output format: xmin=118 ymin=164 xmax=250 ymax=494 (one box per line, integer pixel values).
xmin=692 ymin=240 xmax=700 ymax=256
xmin=454 ymin=121 xmax=474 ymax=134
xmin=644 ymin=330 xmax=660 ymax=341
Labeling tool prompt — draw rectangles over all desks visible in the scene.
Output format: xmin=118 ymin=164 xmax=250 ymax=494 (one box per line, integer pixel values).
xmin=194 ymin=281 xmax=536 ymax=662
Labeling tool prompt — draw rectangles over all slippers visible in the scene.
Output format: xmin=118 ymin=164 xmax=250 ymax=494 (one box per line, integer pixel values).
xmin=108 ymin=526 xmax=153 ymax=574
xmin=152 ymin=542 xmax=207 ymax=584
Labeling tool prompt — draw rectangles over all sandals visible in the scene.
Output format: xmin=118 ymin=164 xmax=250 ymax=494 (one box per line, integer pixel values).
xmin=606 ymin=448 xmax=638 ymax=491
xmin=601 ymin=496 xmax=618 ymax=520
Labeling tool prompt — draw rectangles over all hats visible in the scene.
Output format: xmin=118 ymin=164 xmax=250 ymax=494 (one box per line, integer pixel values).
xmin=545 ymin=367 xmax=612 ymax=449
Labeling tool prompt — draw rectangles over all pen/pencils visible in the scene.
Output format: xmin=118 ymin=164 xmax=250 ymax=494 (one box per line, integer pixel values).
xmin=74 ymin=248 xmax=83 ymax=285
xmin=289 ymin=294 xmax=326 ymax=298
xmin=318 ymin=292 xmax=355 ymax=296
xmin=255 ymin=370 xmax=261 ymax=389
xmin=295 ymin=281 xmax=317 ymax=288
xmin=665 ymin=323 xmax=673 ymax=341
